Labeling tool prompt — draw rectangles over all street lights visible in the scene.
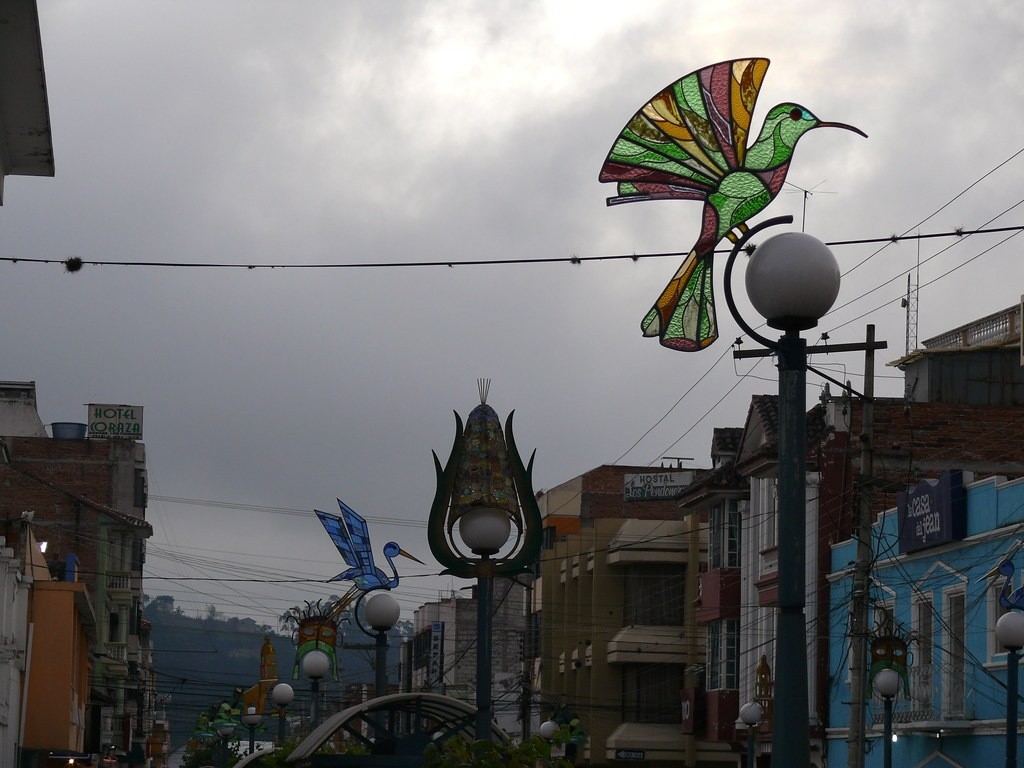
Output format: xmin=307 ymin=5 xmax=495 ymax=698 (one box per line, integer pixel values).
xmin=739 ymin=701 xmax=762 ymax=768
xmin=995 ymin=611 xmax=1024 ymax=768
xmin=273 ymin=684 xmax=295 ymax=748
xmin=539 ymin=720 xmax=557 ymax=759
xmin=745 ymin=231 xmax=842 ymax=768
xmin=303 ymin=650 xmax=330 ymax=733
xmin=458 ymin=507 xmax=511 ymax=768
xmin=244 ymin=715 xmax=261 ymax=755
xmin=365 ymin=594 xmax=401 ymax=738
xmin=874 ymin=667 xmax=903 ymax=768
xmin=220 ymin=728 xmax=234 ymax=768
